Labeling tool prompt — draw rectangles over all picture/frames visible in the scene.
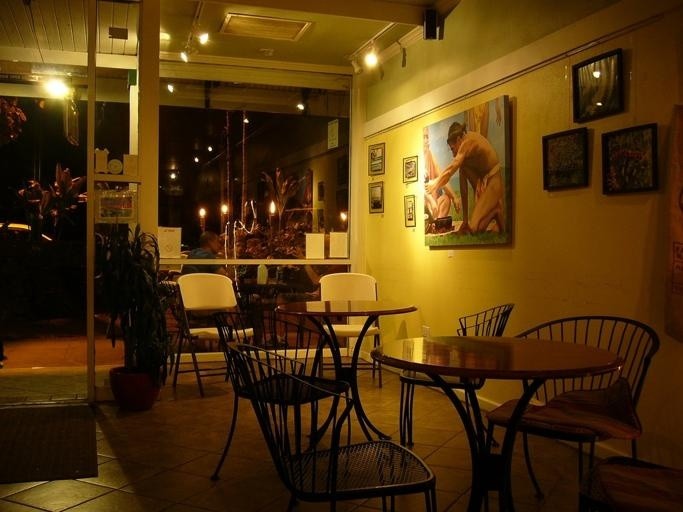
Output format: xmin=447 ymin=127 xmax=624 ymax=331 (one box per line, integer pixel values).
xmin=368 ymin=142 xmax=385 ymax=176
xmin=404 ymin=195 xmax=416 ymax=227
xmin=601 ymin=122 xmax=661 ymax=195
xmin=368 ymin=181 xmax=384 ymax=213
xmin=403 ymin=155 xmax=418 ymax=183
xmin=572 ymin=47 xmax=625 ymax=123
xmin=542 ymin=126 xmax=590 ymax=190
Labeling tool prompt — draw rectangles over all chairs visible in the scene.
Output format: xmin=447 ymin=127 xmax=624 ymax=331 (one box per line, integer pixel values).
xmin=318 ymin=273 xmax=382 ymax=389
xmin=485 ymin=316 xmax=660 ymax=512
xmin=399 ymin=303 xmax=514 ymax=447
xmin=227 ymin=342 xmax=437 ymax=512
xmin=211 ymin=312 xmax=350 ymax=481
xmin=174 ymin=274 xmax=253 ymax=398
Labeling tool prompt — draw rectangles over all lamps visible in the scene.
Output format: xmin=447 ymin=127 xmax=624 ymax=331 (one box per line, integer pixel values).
xmin=347 ymin=23 xmax=401 ymax=75
xmin=180 ymin=0 xmax=210 ymax=63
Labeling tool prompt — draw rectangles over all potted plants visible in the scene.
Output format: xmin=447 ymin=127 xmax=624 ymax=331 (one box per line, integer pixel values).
xmin=94 ymin=225 xmax=177 ymax=410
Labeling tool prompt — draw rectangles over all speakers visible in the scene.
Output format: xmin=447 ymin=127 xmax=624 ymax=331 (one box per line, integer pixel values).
xmin=425 ymin=12 xmax=436 ymax=40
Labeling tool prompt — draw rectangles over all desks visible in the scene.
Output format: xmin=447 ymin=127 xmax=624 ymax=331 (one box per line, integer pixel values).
xmin=275 ymin=300 xmax=419 ymax=449
xmin=371 ymin=336 xmax=624 ymax=512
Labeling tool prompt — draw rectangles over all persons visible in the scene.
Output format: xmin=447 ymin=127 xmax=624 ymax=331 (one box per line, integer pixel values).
xmin=424 ymin=131 xmax=460 ymax=232
xmin=427 ymin=122 xmax=505 ymax=234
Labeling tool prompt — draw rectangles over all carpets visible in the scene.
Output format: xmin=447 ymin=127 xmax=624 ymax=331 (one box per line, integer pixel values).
xmin=0 ymin=406 xmax=98 ymax=484
